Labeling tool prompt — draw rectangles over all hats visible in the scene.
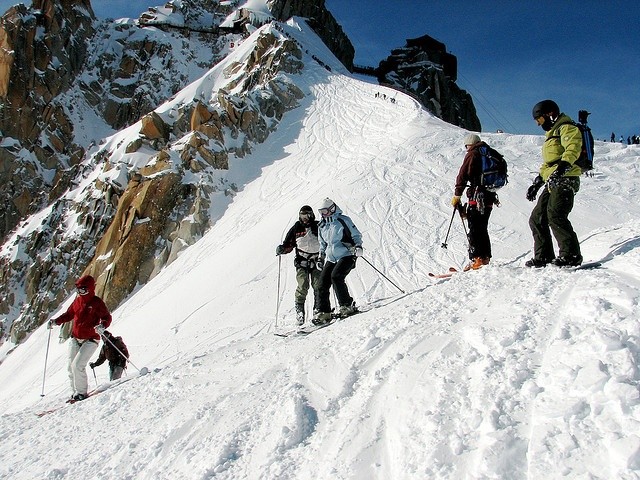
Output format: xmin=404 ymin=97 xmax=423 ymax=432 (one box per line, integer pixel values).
xmin=463 ymin=134 xmax=480 ymax=147
xmin=101 ymin=331 xmax=110 ymax=340
xmin=318 ymin=197 xmax=335 ymax=212
xmin=299 ymin=204 xmax=314 ymax=214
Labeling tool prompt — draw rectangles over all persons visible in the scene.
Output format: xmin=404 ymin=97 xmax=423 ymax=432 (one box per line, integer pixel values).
xmin=275 ymin=203 xmax=332 ymax=327
xmin=308 ymin=194 xmax=365 ymax=326
xmin=449 ymin=132 xmax=508 ymax=272
xmin=89 ymin=323 xmax=130 ymax=383
xmin=47 ymin=275 xmax=113 ymax=404
xmin=524 ymin=98 xmax=586 ymax=268
xmin=604 ymin=129 xmax=640 ymax=145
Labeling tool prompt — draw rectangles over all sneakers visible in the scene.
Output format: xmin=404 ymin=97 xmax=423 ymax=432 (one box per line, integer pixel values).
xmin=312 ymin=311 xmax=333 ymax=325
xmin=295 ymin=313 xmax=305 ymax=325
xmin=526 ymin=258 xmax=555 ymax=268
xmin=73 ymin=393 xmax=88 ymax=400
xmin=482 ymin=257 xmax=491 ymax=265
xmin=463 ymin=257 xmax=484 ymax=270
xmin=551 ymin=255 xmax=584 ymax=267
xmin=338 ymin=302 xmax=360 ymax=319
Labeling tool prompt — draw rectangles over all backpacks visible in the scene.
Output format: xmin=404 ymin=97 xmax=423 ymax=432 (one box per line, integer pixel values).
xmin=115 ymin=336 xmax=130 ymax=366
xmin=479 ymin=146 xmax=509 ymax=192
xmin=545 ymin=120 xmax=595 ymax=174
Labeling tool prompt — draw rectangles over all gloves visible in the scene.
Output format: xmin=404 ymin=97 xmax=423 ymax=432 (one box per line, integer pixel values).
xmin=89 ymin=363 xmax=95 ymax=368
xmin=546 ymin=170 xmax=561 ymax=190
xmin=46 ymin=319 xmax=56 ymax=330
xmin=525 ymin=184 xmax=538 ymax=202
xmin=351 ymin=246 xmax=363 ymax=257
xmin=276 ymin=245 xmax=285 ymax=256
xmin=452 ymin=198 xmax=461 ymax=207
xmin=316 ymin=259 xmax=324 ymax=272
xmin=94 ymin=323 xmax=105 ymax=335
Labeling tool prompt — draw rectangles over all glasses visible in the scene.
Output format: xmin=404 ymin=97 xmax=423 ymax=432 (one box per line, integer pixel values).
xmin=298 ymin=213 xmax=312 ymax=220
xmin=316 ymin=202 xmax=336 ymax=214
xmin=74 ymin=286 xmax=86 ymax=294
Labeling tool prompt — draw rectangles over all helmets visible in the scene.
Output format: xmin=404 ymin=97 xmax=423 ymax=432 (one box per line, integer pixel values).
xmin=532 ymin=99 xmax=560 ymax=131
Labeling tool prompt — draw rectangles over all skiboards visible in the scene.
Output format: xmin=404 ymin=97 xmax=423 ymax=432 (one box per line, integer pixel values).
xmin=428 ymin=267 xmax=459 ymax=278
xmin=34 ymin=379 xmax=129 ymax=419
xmin=517 ymin=262 xmax=602 ymax=272
xmin=273 ymin=307 xmax=335 ymax=337
